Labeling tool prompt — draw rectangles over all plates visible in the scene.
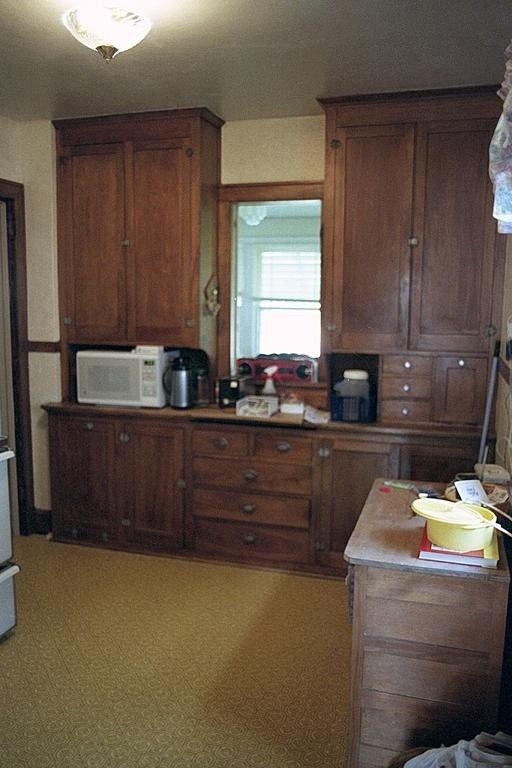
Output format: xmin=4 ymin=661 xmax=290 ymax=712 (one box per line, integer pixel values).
xmin=52 ymin=106 xmax=226 ymax=345
xmin=41 ymin=405 xmax=186 ymax=561
xmin=188 ymin=414 xmax=316 ymax=577
xmin=344 ymin=474 xmax=512 ymax=766
xmin=317 ymin=422 xmax=498 ymax=584
xmin=0 ymin=449 xmax=19 ymax=639
xmin=314 ymin=83 xmax=511 ymax=352
xmin=377 ymin=351 xmax=497 ymax=431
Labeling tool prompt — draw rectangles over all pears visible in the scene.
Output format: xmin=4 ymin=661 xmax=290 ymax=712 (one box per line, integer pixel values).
xmin=260 ymin=365 xmax=280 ymax=396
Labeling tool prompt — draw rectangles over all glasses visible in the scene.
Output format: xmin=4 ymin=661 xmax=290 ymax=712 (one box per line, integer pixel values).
xmin=237 ymin=204 xmax=268 ymax=226
xmin=58 ymin=1 xmax=157 ymax=60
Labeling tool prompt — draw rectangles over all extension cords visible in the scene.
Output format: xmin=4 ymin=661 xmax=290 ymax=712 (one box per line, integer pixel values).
xmin=161 ymin=354 xmax=193 ymax=410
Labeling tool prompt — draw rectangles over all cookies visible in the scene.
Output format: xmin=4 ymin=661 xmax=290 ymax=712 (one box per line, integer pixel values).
xmin=74 ymin=347 xmax=182 ymax=407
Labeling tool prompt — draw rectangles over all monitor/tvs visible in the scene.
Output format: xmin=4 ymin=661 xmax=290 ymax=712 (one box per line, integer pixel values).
xmin=416 ymin=518 xmax=500 ymax=569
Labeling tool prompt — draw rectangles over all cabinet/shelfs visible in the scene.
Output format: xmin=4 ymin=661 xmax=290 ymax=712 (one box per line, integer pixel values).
xmin=340 ymin=370 xmax=371 ymax=418
xmin=194 ymin=367 xmax=211 ymax=407
